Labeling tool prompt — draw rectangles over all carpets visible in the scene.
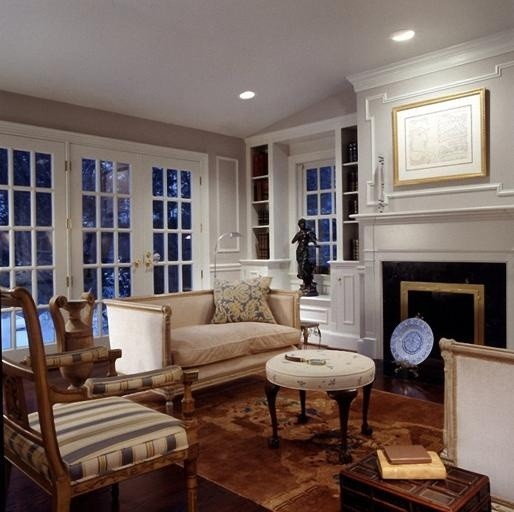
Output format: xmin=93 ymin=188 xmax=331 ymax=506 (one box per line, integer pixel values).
xmin=162 ymin=376 xmax=445 ymax=512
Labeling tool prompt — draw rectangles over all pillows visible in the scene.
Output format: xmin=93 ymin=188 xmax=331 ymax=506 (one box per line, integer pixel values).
xmin=212 ymin=278 xmax=276 ymax=324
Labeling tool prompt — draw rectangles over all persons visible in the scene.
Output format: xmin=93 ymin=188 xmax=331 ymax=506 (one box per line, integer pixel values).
xmin=292 ymin=218 xmax=320 ymax=295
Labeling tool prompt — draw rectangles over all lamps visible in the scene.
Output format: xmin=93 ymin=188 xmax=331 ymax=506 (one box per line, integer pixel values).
xmin=213 ymin=231 xmax=243 ymax=277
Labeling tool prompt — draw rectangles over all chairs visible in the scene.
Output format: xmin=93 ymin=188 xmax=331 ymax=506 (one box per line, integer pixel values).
xmin=47 ymin=291 xmax=183 ymax=402
xmin=0 ymin=285 xmax=201 ymax=512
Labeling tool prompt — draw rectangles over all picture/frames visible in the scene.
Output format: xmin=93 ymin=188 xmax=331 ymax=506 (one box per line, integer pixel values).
xmin=389 ymin=89 xmax=490 ymax=189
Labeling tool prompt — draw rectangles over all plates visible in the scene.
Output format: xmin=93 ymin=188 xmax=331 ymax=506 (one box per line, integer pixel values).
xmin=391 ymin=316 xmax=435 ymax=366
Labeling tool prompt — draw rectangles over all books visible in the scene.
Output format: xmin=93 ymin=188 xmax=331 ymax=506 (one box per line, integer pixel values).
xmin=375 ymin=448 xmax=447 ymax=480
xmin=383 ymin=445 xmax=431 ymax=464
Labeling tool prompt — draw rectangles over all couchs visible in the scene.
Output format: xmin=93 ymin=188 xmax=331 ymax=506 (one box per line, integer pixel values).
xmin=100 ymin=287 xmax=300 ymax=402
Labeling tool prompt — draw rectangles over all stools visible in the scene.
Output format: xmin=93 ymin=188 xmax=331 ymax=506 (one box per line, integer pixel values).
xmin=301 ymin=320 xmax=322 ymax=348
xmin=336 ymin=451 xmax=492 ymax=512
